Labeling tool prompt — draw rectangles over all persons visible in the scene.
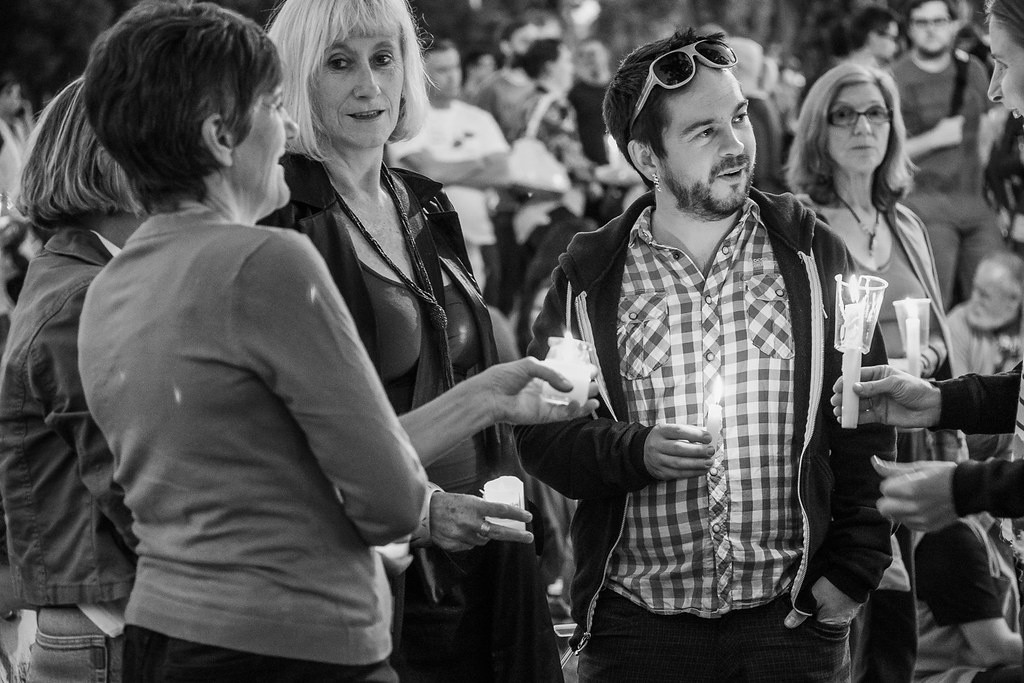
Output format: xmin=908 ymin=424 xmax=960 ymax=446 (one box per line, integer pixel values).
xmin=83 ymin=0 xmax=601 ymax=683
xmin=1 ymin=2 xmax=649 ymax=627
xmin=251 ymin=1 xmax=566 ymax=682
xmin=832 ymin=0 xmax=1023 ymax=534
xmin=723 ymin=2 xmax=1024 ymax=683
xmin=513 ymin=26 xmax=900 ymax=683
xmin=786 ymin=63 xmax=963 ymax=683
xmin=0 ymin=74 xmax=154 ymax=682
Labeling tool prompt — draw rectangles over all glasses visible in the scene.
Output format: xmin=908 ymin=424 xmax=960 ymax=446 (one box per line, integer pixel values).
xmin=828 ymin=104 xmax=892 ymax=127
xmin=882 ymin=30 xmax=897 ymax=40
xmin=912 ymin=18 xmax=949 ymax=28
xmin=631 ymin=36 xmax=739 ymax=139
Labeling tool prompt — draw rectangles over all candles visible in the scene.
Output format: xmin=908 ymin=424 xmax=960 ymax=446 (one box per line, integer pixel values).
xmin=905 ymin=296 xmax=920 ymax=377
xmin=839 ymin=273 xmax=867 ymax=429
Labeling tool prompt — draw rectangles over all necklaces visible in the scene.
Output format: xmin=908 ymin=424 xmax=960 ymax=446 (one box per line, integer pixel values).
xmin=835 ymin=194 xmax=881 ymax=258
xmin=314 ymin=155 xmax=455 ymax=392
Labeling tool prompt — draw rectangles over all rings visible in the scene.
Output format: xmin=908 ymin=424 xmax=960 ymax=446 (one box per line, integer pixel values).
xmin=868 ymin=398 xmax=872 ymax=408
xmin=481 ymin=521 xmax=493 ymax=535
